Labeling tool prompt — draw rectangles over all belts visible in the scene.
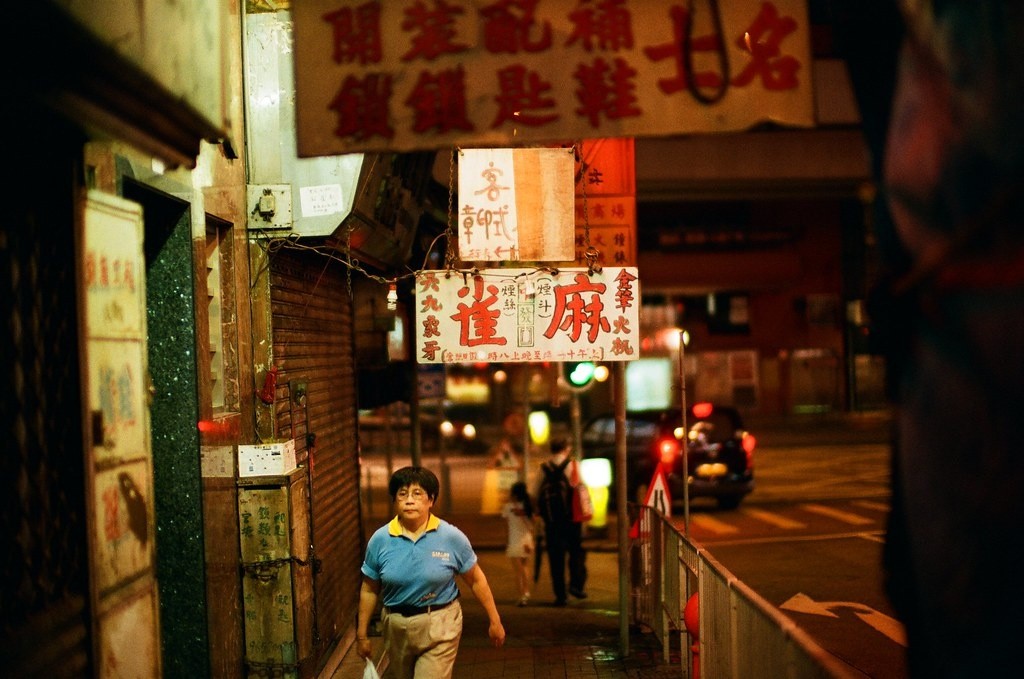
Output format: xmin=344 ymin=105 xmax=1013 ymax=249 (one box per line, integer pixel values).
xmin=386 ymin=596 xmax=458 ymax=617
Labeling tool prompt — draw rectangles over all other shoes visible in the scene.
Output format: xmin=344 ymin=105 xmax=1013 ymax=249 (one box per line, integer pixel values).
xmin=569 ymin=588 xmax=588 ymax=599
xmin=553 ymin=598 xmax=568 ymax=607
xmin=517 ymin=591 xmax=531 ymax=606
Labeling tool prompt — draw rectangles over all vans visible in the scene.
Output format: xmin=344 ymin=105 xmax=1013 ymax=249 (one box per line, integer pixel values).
xmin=582 ymin=403 xmax=754 ymax=513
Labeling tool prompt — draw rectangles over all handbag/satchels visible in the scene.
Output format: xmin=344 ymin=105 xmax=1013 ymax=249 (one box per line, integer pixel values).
xmin=363 ymin=656 xmax=379 ymax=679
xmin=570 ymin=461 xmax=592 ymax=523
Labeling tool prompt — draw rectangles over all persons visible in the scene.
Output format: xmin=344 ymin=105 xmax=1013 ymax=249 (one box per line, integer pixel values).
xmin=533 ymin=438 xmax=589 ymax=605
xmin=501 ymin=481 xmax=543 ymax=605
xmin=355 ymin=467 xmax=505 ymax=679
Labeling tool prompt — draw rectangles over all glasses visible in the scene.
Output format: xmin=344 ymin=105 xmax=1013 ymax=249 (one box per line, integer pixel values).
xmin=397 ymin=489 xmax=429 ymax=501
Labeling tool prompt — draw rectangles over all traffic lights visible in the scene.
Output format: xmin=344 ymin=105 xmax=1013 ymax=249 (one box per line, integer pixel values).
xmin=558 ymin=362 xmax=596 ymax=393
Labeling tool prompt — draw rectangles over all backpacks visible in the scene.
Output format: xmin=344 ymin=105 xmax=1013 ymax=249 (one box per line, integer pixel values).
xmin=538 ymin=458 xmax=573 ymax=523
